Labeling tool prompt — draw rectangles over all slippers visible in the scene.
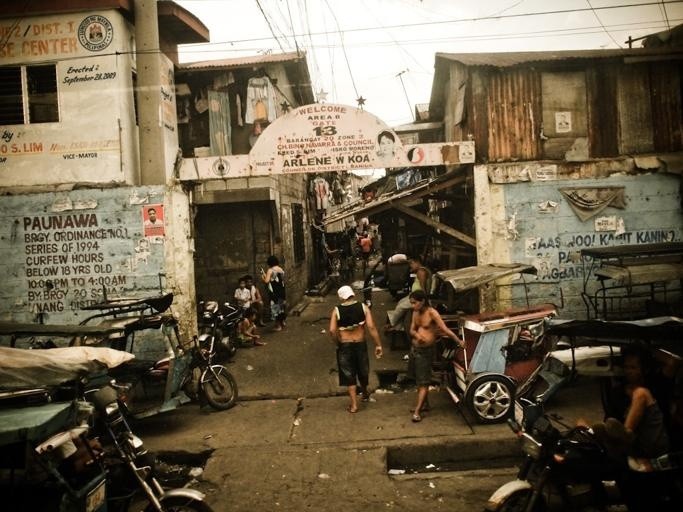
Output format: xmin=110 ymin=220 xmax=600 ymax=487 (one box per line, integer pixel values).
xmin=410 ymin=405 xmax=431 ymax=422
xmin=345 ymin=391 xmax=371 ymax=413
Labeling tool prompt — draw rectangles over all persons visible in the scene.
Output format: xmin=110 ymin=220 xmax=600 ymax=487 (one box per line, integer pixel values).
xmin=351 ymin=222 xmax=385 ymax=275
xmin=233 ymin=278 xmax=249 ymax=322
xmin=242 ymin=272 xmax=266 ymax=329
xmin=259 ymin=253 xmax=286 ymax=332
xmin=600 ymin=341 xmax=668 ymax=463
xmin=382 ymin=254 xmax=434 ymax=335
xmin=236 ymin=312 xmax=266 ymax=347
xmin=329 ymin=285 xmax=385 ymax=413
xmin=404 ymin=289 xmax=463 ymax=422
xmin=142 ymin=207 xmax=164 ymax=225
xmin=372 ymin=130 xmax=401 ymax=165
